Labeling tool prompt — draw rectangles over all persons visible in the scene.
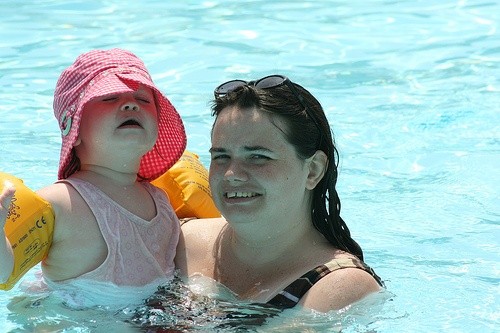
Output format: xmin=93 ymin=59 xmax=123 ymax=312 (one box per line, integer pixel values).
xmin=0 ymin=48 xmax=188 ymax=290
xmin=173 ymin=74 xmax=385 ymax=314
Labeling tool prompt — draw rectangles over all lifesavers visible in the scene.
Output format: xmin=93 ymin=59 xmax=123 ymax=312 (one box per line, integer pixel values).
xmin=153 ymin=150 xmax=222 ymax=218
xmin=0 ymin=174 xmax=55 ymax=291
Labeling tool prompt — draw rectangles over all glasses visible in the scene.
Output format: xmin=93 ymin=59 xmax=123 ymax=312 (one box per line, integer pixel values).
xmin=214 ymin=74 xmax=326 ymax=139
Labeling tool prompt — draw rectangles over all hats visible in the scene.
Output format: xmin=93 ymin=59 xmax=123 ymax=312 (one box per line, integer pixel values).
xmin=53 ymin=47 xmax=187 ymax=182
